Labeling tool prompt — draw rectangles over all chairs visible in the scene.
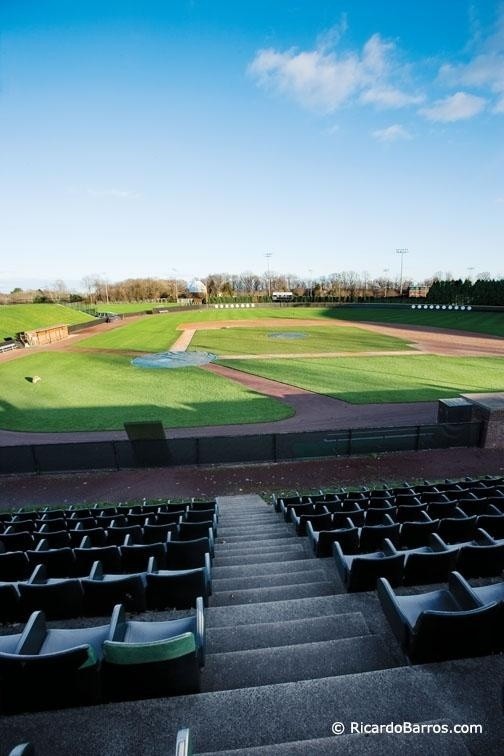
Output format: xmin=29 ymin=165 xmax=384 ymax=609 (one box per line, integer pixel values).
xmin=0 ymin=495 xmax=220 ymax=712
xmin=256 ymin=475 xmax=504 ymax=665
